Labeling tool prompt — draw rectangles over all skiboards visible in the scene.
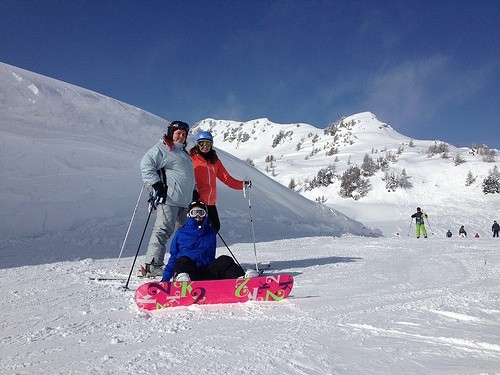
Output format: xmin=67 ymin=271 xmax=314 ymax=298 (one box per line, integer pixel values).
xmin=243 ymin=264 xmax=270 ymax=270
xmin=89 ymin=277 xmax=128 ymax=281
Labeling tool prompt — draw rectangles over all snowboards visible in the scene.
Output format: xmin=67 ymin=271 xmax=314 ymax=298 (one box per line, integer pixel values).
xmin=135 ymin=274 xmax=293 ymax=311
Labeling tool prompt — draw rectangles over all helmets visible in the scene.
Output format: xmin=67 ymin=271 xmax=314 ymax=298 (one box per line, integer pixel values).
xmin=187 ymin=201 xmax=208 ymax=218
xmin=196 ymin=132 xmax=213 ymax=150
xmin=168 ymin=121 xmax=189 ymax=142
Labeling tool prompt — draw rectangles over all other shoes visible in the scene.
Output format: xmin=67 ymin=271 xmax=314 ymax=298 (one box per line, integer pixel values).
xmin=141 ymin=264 xmax=164 ymax=277
xmin=244 ymin=269 xmax=260 ymax=279
xmin=175 ymin=273 xmax=190 ymax=282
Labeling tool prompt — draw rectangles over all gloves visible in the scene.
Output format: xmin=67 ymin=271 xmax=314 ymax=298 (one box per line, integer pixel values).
xmin=193 ymin=190 xmax=200 ymax=202
xmin=245 ymin=180 xmax=252 ymax=188
xmin=150 ymin=181 xmax=166 ymax=204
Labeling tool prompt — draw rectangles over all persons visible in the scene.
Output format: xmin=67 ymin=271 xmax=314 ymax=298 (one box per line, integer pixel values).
xmin=446 ymin=230 xmax=452 ymax=238
xmin=491 ymin=220 xmax=500 ymax=237
xmin=159 ymin=200 xmax=260 ymax=283
xmin=458 ymin=225 xmax=467 ymax=238
xmin=411 ymin=207 xmax=428 ymax=238
xmin=187 ymin=131 xmax=252 ymax=234
xmin=137 ymin=121 xmax=200 ymax=277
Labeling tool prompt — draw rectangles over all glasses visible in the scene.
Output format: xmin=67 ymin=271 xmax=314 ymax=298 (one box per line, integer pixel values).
xmin=199 ymin=141 xmax=212 ymax=147
xmin=172 ymin=122 xmax=189 ymax=131
xmin=189 ymin=208 xmax=207 ymax=217
